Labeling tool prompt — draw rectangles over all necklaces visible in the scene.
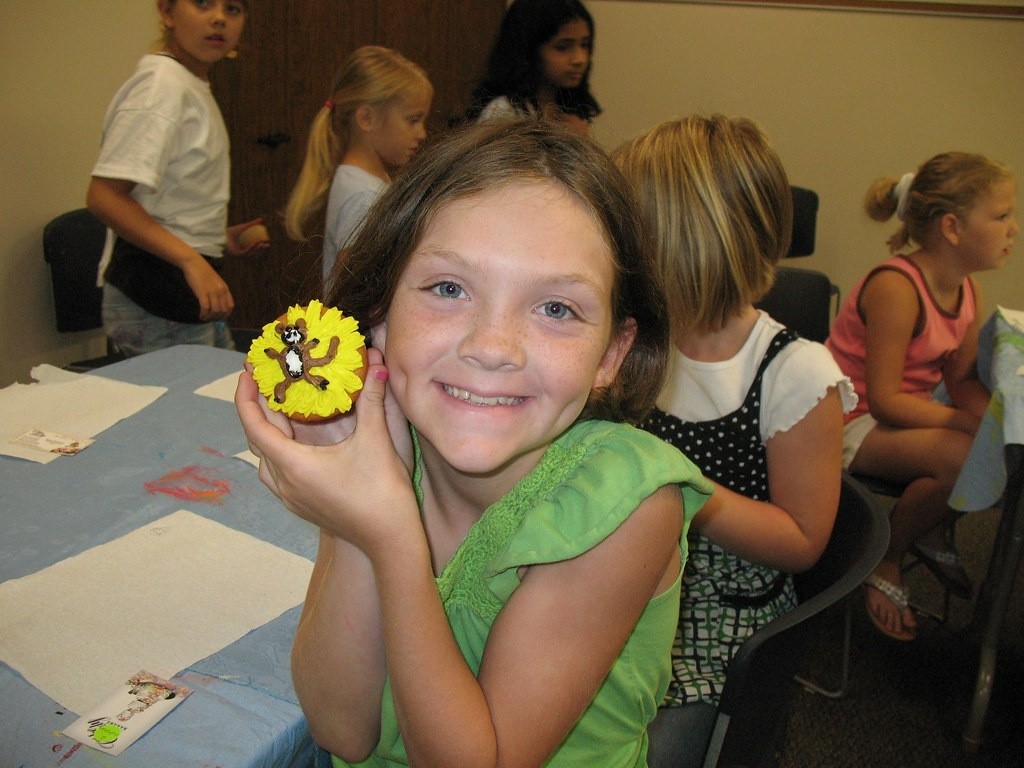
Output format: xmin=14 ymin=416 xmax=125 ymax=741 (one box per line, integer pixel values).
xmin=157 ymin=52 xmax=187 ymax=66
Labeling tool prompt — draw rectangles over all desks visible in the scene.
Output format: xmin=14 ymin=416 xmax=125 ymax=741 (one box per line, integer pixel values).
xmin=931 ymin=304 xmax=1024 ymax=756
xmin=0 ymin=345 xmax=332 ymax=768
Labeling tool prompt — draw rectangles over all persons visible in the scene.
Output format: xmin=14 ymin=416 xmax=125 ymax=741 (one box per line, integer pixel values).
xmin=235 ymin=119 xmax=715 ymax=768
xmin=285 ymin=46 xmax=435 ymax=301
xmin=607 ymin=113 xmax=857 ymax=768
xmin=825 ymin=152 xmax=1020 ymax=640
xmin=451 ymin=0 xmax=601 ymax=134
xmin=85 ymin=0 xmax=270 ymax=350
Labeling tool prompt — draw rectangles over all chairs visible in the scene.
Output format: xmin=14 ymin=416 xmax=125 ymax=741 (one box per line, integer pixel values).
xmin=789 ymin=473 xmax=966 ymax=700
xmin=702 ymin=471 xmax=890 ymax=768
xmin=752 ymin=185 xmax=840 ymax=345
xmin=42 ymin=207 xmax=115 ymax=373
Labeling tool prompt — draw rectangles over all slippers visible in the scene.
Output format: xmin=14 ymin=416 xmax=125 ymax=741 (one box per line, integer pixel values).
xmin=910 ymin=537 xmax=972 ymax=601
xmin=863 ymin=574 xmax=917 ymax=643
